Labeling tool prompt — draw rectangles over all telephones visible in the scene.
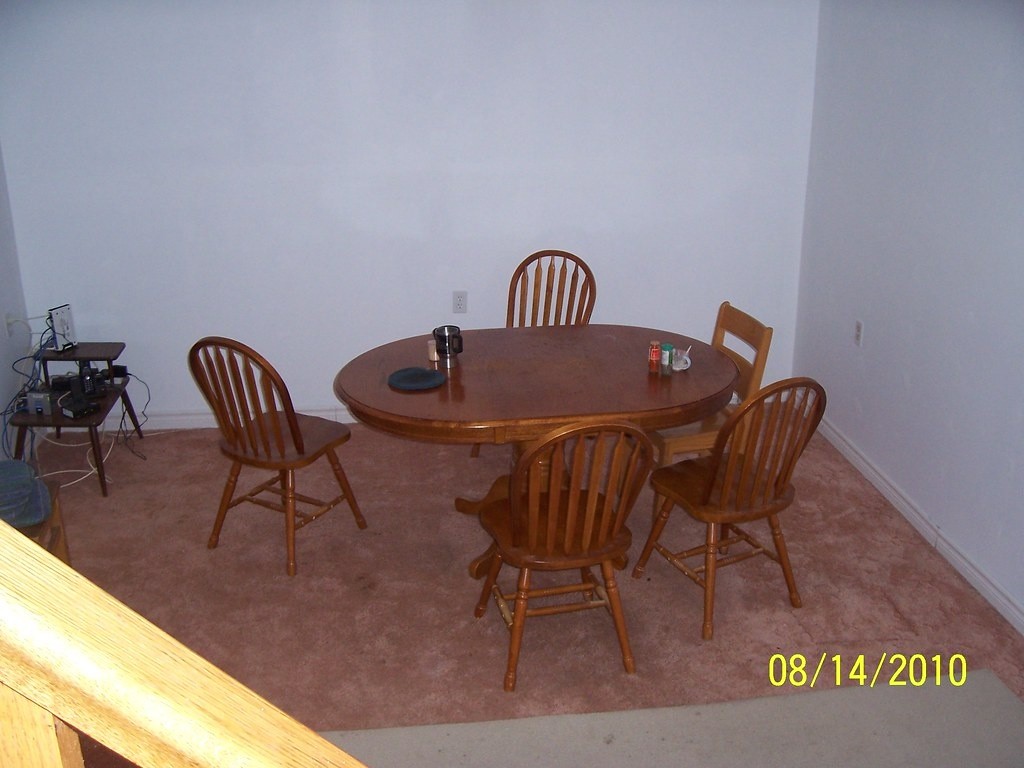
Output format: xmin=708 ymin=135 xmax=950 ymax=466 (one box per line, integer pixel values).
xmin=81 ymin=366 xmax=108 ymax=398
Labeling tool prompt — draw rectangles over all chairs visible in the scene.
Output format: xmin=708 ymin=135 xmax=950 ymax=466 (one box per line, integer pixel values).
xmin=468 ymin=249 xmax=596 ymax=457
xmin=188 ymin=336 xmax=368 ymax=576
xmin=630 ymin=376 xmax=827 ymax=640
xmin=648 ymin=301 xmax=774 ymax=521
xmin=476 ymin=418 xmax=658 ymax=693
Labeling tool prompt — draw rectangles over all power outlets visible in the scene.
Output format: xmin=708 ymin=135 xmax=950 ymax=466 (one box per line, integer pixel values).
xmin=452 ymin=290 xmax=467 ymax=314
xmin=4 ymin=312 xmax=16 ymax=340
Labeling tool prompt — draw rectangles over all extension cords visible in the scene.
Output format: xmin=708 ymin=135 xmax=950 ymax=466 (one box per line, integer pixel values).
xmin=104 ymin=376 xmax=123 ymax=384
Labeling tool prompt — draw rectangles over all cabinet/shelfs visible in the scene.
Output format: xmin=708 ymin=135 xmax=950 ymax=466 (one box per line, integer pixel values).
xmin=8 ymin=342 xmax=144 ymax=498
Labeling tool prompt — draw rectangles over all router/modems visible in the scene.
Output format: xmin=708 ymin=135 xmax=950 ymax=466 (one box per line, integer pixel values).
xmin=49 ymin=302 xmax=78 ymax=351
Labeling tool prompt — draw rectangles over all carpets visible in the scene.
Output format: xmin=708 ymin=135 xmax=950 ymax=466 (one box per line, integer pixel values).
xmin=322 ymin=669 xmax=1024 ymax=768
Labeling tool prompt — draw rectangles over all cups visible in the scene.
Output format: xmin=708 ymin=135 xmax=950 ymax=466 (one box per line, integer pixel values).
xmin=432 ymin=325 xmax=463 ymax=368
xmin=427 ymin=339 xmax=440 ymax=361
xmin=671 ymin=349 xmax=691 ymax=372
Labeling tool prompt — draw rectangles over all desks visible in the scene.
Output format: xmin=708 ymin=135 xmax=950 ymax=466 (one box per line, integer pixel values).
xmin=17 ymin=480 xmax=70 ymax=569
xmin=332 ymin=323 xmax=740 ymax=580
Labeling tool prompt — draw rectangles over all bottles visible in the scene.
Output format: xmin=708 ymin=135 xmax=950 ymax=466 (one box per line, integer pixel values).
xmin=647 ymin=340 xmax=661 ymax=374
xmin=660 ymin=343 xmax=673 ymax=376
